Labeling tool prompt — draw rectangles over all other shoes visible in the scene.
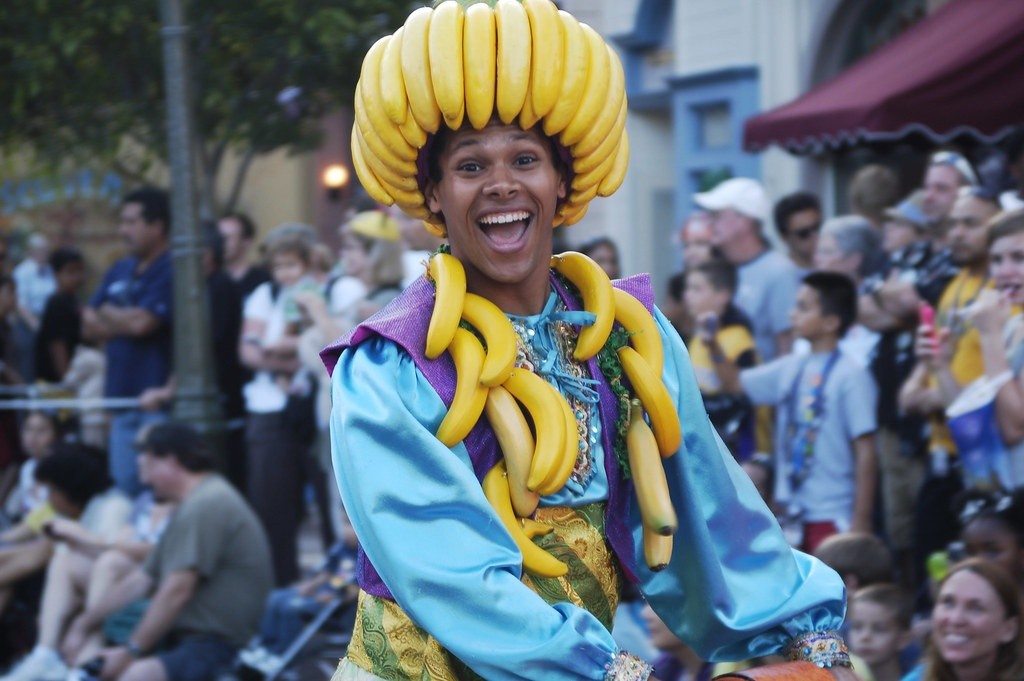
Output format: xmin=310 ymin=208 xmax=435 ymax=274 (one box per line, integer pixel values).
xmin=0 ymin=646 xmax=71 ymax=681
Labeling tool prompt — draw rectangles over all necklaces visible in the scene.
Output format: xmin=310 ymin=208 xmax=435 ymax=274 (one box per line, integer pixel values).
xmin=775 ymin=349 xmax=839 ymax=486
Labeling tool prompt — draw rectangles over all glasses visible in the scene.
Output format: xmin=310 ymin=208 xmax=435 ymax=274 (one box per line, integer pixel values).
xmin=790 ymin=224 xmax=824 ymax=240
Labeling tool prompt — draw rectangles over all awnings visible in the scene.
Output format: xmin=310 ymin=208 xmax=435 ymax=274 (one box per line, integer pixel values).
xmin=745 ymin=2 xmax=1024 ymax=149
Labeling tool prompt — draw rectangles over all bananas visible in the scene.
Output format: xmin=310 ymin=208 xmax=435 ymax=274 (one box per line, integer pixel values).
xmin=425 ymin=252 xmax=683 ymax=577
xmin=349 ymin=0 xmax=627 ymax=229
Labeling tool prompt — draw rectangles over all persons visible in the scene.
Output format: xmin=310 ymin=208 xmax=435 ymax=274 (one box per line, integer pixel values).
xmin=0 ymin=189 xmax=439 ymax=681
xmin=317 ymin=0 xmax=857 ymax=681
xmin=582 ymin=151 xmax=1024 ymax=680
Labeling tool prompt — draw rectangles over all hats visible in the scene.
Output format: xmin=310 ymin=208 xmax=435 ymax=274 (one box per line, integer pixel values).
xmin=350 ymin=1 xmax=630 ymax=237
xmin=880 ymin=195 xmax=932 ymax=231
xmin=694 ymin=176 xmax=775 ymax=222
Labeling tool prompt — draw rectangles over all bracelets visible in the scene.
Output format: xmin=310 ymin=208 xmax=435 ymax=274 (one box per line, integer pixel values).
xmin=126 ymin=641 xmax=142 ymax=658
